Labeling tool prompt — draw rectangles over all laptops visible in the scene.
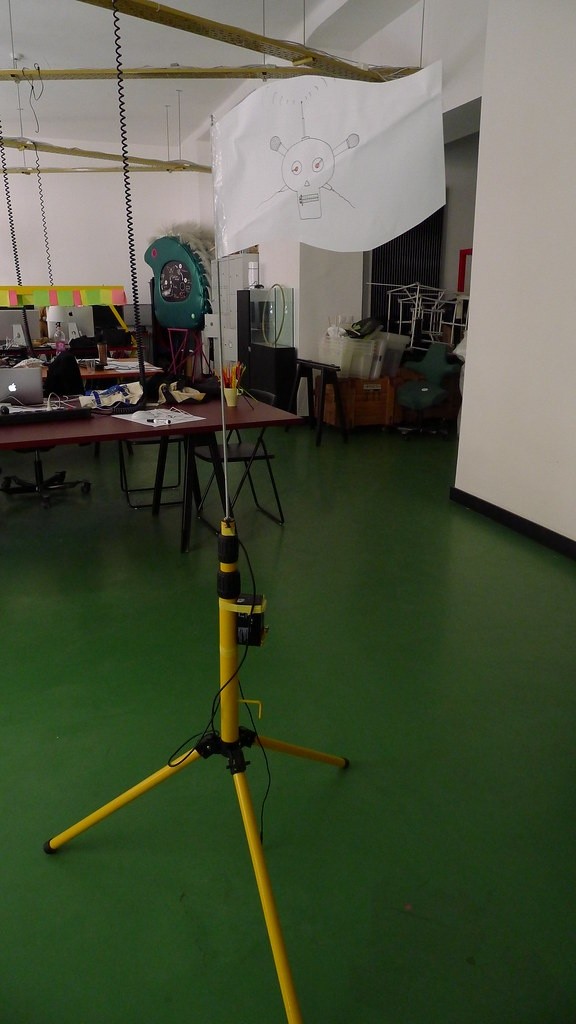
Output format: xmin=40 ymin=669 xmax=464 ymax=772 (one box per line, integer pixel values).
xmin=0 ymin=366 xmax=43 ymax=405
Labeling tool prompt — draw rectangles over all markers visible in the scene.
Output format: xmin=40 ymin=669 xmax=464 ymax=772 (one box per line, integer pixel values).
xmin=147 ymin=419 xmax=171 ymax=424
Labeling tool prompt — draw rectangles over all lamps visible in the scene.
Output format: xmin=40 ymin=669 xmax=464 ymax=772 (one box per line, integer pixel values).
xmin=46 ymin=305 xmax=67 ymax=342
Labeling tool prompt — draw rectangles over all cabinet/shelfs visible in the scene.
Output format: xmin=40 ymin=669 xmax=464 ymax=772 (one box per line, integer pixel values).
xmin=234 ymin=288 xmax=297 ymax=412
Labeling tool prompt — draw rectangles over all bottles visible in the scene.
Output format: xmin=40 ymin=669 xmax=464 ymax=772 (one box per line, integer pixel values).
xmin=54 ymin=327 xmax=66 ymax=357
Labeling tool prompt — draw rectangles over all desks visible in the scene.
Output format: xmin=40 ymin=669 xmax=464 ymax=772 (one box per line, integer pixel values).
xmin=36 ymin=357 xmax=165 ymax=393
xmin=0 ymin=389 xmax=304 ymax=555
xmin=29 ymin=347 xmax=146 ymax=359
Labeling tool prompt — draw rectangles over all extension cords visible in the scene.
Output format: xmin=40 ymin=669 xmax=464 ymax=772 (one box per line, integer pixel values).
xmin=0 ymin=405 xmax=92 ymax=428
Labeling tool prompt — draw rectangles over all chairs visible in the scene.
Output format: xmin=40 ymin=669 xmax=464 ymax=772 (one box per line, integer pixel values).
xmin=180 ymin=390 xmax=284 ymax=536
xmin=0 ymin=353 xmax=90 ymax=511
xmin=118 ymin=374 xmax=186 ymax=510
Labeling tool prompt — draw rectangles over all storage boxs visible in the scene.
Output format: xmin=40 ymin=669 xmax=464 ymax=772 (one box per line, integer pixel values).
xmin=322 ymin=331 xmax=410 ymax=380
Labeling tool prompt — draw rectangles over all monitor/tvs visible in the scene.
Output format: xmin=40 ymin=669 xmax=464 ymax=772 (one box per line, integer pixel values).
xmin=0 ymin=309 xmax=42 ymax=345
xmin=47 ymin=305 xmax=95 ymax=341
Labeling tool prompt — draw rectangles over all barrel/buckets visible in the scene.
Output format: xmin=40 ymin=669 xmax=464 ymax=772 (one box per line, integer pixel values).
xmin=380 ymin=331 xmax=410 ymax=378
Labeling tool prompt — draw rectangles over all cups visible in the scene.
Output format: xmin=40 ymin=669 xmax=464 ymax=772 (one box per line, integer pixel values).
xmin=97 ymin=342 xmax=108 ymax=363
xmin=223 ymin=388 xmax=243 ymax=407
